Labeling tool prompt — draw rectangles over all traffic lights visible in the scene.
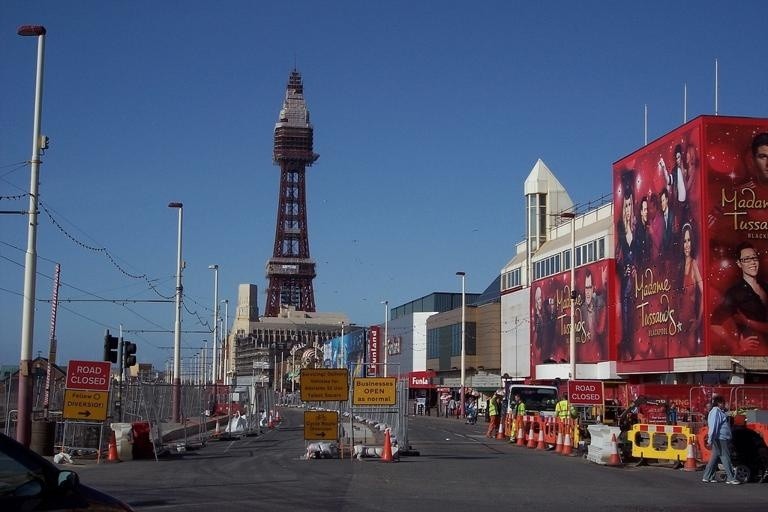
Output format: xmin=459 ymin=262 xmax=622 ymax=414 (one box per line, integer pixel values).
xmin=108 ymin=335 xmax=119 ymax=364
xmin=127 ymin=342 xmax=137 ymax=367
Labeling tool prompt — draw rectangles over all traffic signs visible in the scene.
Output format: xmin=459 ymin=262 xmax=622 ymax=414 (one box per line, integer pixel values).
xmin=64 ymin=389 xmax=110 ymax=421
xmin=305 ymin=408 xmax=339 ymax=440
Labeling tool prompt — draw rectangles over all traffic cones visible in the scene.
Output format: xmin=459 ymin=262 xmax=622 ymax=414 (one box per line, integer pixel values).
xmin=561 ymin=427 xmax=574 ymax=455
xmin=379 ymin=430 xmax=394 ymax=461
xmin=605 ymin=433 xmax=626 ymax=467
xmin=275 ymin=411 xmax=279 ymax=422
xmin=534 ymin=425 xmax=547 ymax=452
xmin=526 ymin=424 xmax=537 ymax=448
xmin=212 ymin=419 xmax=223 ymax=436
xmin=105 ymin=432 xmax=121 ymax=464
xmin=550 ymin=425 xmax=563 ymax=454
xmin=515 ymin=422 xmax=525 ymax=448
xmin=680 ymin=436 xmax=702 ymax=472
xmin=268 ymin=414 xmax=274 ymax=428
xmin=495 ymin=417 xmax=506 ymax=440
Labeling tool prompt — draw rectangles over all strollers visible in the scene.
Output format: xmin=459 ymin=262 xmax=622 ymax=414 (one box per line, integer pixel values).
xmin=465 ymin=407 xmax=477 ymax=425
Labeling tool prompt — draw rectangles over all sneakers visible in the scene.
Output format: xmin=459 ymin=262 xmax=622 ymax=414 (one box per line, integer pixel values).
xmin=701 ymin=478 xmax=718 ymax=483
xmin=725 ymin=479 xmax=740 ymax=485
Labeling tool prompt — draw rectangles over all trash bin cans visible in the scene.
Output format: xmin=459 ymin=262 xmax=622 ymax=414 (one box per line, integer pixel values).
xmin=111 ymin=421 xmax=153 ymax=461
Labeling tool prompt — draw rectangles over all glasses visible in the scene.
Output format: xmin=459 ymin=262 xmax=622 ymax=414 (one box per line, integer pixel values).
xmin=739 ymin=257 xmax=761 ymax=263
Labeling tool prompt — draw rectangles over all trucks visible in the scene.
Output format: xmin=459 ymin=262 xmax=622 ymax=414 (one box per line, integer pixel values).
xmin=592 ymin=398 xmax=620 ymax=424
xmin=507 ymin=384 xmax=559 ymax=423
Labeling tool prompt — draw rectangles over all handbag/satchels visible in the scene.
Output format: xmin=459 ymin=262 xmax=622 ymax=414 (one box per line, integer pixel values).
xmin=703 ymin=434 xmax=711 ymax=450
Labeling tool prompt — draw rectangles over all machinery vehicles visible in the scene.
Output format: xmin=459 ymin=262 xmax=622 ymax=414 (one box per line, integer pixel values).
xmin=696 ymin=410 xmax=768 ymax=483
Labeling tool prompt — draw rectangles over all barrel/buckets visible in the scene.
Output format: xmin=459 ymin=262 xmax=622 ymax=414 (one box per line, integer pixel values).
xmin=29 ymin=421 xmax=56 ymax=456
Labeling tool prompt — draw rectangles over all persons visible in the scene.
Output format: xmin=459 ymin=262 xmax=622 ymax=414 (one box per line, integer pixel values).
xmin=617 ymin=143 xmax=703 ymax=360
xmin=507 ymin=394 xmax=525 ymax=444
xmin=577 ymin=269 xmax=605 ymax=363
xmin=554 ymin=394 xmax=577 ymax=448
xmin=447 ymin=399 xmax=477 ymax=425
xmin=666 ymin=402 xmax=677 ymax=425
xmin=485 ymin=393 xmax=502 ymax=438
xmin=702 ymin=394 xmax=742 ymax=485
xmin=531 ymin=285 xmax=543 ymax=366
xmin=741 ymin=133 xmax=768 ymax=225
xmin=710 ymin=243 xmax=768 ymax=356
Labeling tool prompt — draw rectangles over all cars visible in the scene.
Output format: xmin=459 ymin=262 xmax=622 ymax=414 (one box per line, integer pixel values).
xmin=1 ymin=431 xmax=133 ymax=512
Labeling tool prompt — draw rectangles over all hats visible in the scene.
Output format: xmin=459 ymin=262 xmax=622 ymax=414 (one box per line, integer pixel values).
xmin=621 ymin=171 xmax=632 ymax=199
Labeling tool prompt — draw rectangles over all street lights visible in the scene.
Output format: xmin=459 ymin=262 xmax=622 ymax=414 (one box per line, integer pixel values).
xmin=168 ymin=200 xmax=183 ymax=421
xmin=217 ymin=319 xmax=223 ymax=380
xmin=16 ymin=22 xmax=48 ymax=448
xmin=337 ymin=320 xmax=344 ymax=368
xmin=559 ymin=211 xmax=577 ymax=380
xmin=312 ymin=330 xmax=319 ymax=368
xmin=379 ymin=300 xmax=389 ymax=377
xmin=208 ymin=263 xmax=220 ymax=387
xmin=455 ymin=270 xmax=467 ymax=417
xmin=221 ymin=299 xmax=229 ymax=385
xmin=163 ymin=339 xmax=208 ymax=391
